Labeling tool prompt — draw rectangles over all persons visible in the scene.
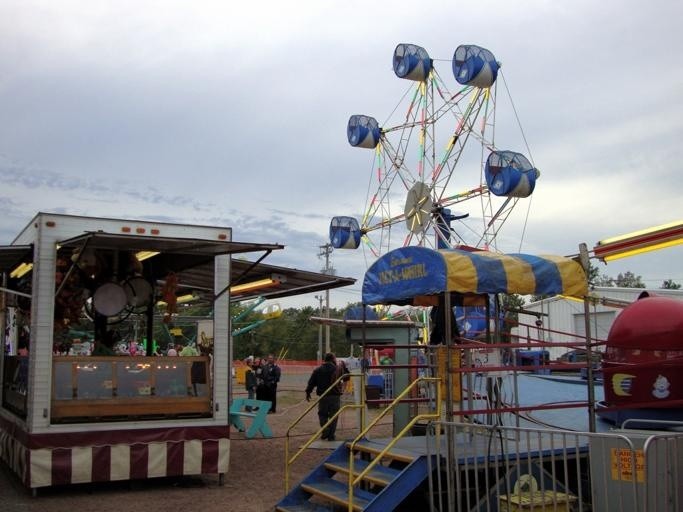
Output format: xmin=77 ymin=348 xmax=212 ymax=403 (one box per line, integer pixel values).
xmin=428 ymin=293 xmax=462 ymax=346
xmin=243 ymin=348 xmax=393 ymax=441
xmin=4 ymin=332 xmax=200 ymax=357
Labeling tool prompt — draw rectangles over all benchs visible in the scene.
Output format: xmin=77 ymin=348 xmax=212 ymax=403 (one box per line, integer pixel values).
xmin=229 ymin=398 xmax=273 ymax=439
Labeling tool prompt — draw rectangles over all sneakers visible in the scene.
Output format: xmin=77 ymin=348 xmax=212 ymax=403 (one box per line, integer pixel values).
xmin=320 ymin=431 xmax=329 ymax=439
xmin=327 ymin=433 xmax=336 ymax=441
xmin=253 ymin=407 xmax=278 ymax=415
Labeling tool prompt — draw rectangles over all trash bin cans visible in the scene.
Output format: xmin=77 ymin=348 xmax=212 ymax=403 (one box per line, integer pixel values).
xmin=365 ymin=385 xmax=380 ymax=409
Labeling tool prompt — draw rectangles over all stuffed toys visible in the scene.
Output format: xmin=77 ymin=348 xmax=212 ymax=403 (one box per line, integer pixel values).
xmin=52 ymin=244 xmax=180 ymax=336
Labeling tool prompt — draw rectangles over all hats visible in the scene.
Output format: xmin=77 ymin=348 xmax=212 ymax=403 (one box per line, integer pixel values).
xmin=324 ymin=352 xmax=336 ymax=361
